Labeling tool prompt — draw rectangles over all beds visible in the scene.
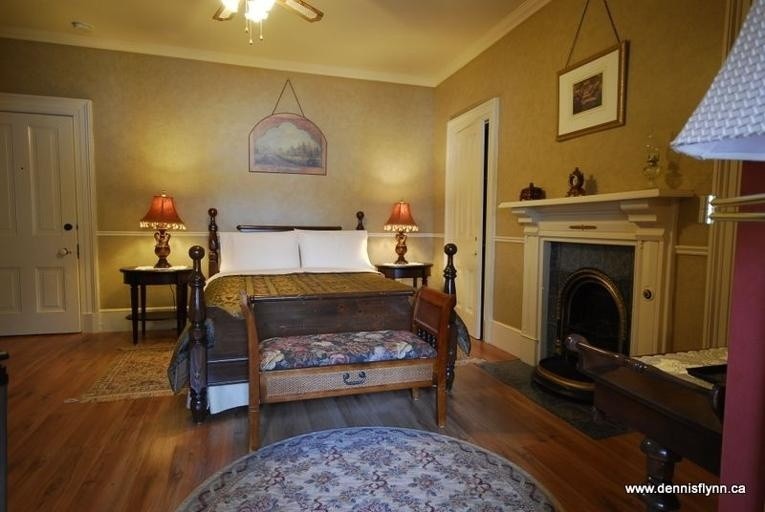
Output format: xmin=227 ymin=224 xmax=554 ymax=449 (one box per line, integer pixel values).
xmin=165 ymin=210 xmax=474 ymax=456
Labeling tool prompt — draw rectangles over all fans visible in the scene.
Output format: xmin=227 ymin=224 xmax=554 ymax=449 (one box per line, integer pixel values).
xmin=211 ymin=0 xmax=325 ymax=27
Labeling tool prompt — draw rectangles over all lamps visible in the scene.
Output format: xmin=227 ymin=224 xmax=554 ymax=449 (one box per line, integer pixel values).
xmin=383 ymin=196 xmax=419 ymax=267
xmin=137 ymin=183 xmax=187 ymax=268
xmin=219 ymin=0 xmax=280 ymax=50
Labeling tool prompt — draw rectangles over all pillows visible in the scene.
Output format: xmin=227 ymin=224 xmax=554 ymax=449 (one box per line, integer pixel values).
xmin=212 ymin=228 xmax=302 ymax=276
xmin=294 ymin=227 xmax=380 ymax=276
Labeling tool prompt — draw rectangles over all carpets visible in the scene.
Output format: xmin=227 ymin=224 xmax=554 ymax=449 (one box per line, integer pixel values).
xmin=80 ymin=341 xmax=186 ymax=404
xmin=166 ymin=425 xmax=562 ymax=511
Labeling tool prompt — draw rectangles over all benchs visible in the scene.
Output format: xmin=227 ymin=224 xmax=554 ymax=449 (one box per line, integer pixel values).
xmin=237 ymin=285 xmax=458 ymax=452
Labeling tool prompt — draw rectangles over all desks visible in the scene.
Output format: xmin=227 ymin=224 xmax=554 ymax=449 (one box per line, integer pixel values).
xmin=592 ymin=364 xmax=726 ymax=512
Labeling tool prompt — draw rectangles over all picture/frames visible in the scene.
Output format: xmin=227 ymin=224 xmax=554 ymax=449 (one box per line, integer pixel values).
xmin=553 ymin=37 xmax=628 ymax=141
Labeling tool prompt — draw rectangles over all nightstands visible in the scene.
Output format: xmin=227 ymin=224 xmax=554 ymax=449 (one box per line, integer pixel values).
xmin=118 ymin=265 xmax=193 ymax=346
xmin=373 ymin=263 xmax=433 ymax=289
